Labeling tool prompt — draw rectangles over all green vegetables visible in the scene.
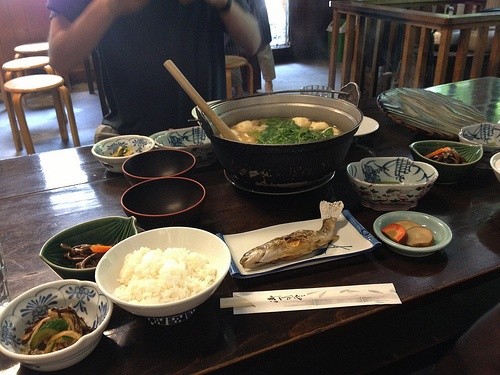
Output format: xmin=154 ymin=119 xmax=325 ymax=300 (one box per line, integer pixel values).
xmin=250 ymin=117 xmax=335 ymax=144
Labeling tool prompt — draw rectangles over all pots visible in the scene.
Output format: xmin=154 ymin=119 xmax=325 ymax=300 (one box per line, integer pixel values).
xmin=196 ymin=82 xmax=364 ymax=196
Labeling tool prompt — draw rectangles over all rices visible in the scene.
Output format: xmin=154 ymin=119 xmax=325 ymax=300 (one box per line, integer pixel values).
xmin=114 ymin=247 xmax=218 ymax=306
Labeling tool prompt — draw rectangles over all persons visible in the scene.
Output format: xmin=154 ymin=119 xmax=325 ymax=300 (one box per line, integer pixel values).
xmin=221 ymin=0 xmax=275 ymax=94
xmin=47 ymin=0 xmax=261 ymax=143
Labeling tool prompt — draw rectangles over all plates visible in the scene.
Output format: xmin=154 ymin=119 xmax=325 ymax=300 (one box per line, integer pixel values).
xmin=354 ymin=116 xmax=379 ymax=136
xmin=215 ymin=210 xmax=383 ymax=278
xmin=373 ymin=211 xmax=454 ymax=257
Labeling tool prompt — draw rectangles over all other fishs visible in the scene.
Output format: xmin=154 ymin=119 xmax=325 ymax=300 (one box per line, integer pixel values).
xmin=239 ymin=200 xmax=344 ymax=269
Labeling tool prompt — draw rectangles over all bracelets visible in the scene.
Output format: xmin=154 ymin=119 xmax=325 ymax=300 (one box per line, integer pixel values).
xmin=215 ymin=0 xmax=232 ymax=13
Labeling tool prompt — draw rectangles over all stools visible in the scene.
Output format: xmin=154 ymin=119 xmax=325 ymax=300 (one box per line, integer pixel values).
xmin=4 ymin=74 xmax=80 ymax=156
xmin=0 ymin=56 xmax=69 ymax=152
xmin=14 ymin=41 xmax=71 ymax=110
xmin=225 ymin=54 xmax=254 ymax=100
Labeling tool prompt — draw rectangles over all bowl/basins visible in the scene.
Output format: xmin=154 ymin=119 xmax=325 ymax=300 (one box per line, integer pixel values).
xmin=90 ymin=135 xmax=155 ymax=172
xmin=120 ymin=177 xmax=205 ymax=230
xmin=0 ymin=279 xmax=113 ymax=371
xmin=95 ymin=227 xmax=231 ymax=326
xmin=459 ymin=122 xmax=500 ymax=169
xmin=346 ymin=156 xmax=439 ymax=210
xmin=38 ymin=216 xmax=138 ymax=283
xmin=191 ymin=100 xmax=222 ymax=125
xmin=155 ymin=126 xmax=216 ymax=157
xmin=408 ymin=140 xmax=483 ymax=183
xmin=121 ymin=149 xmax=195 ymax=186
xmin=490 ymin=152 xmax=500 ymax=181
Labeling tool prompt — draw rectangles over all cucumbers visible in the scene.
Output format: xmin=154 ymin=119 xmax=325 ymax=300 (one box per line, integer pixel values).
xmin=30 ymin=319 xmax=69 ymax=350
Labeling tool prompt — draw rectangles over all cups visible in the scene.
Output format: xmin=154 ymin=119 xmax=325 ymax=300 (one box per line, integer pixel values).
xmin=301 ymin=85 xmax=331 ymax=97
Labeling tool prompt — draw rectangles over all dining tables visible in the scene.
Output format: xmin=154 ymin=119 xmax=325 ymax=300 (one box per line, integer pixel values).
xmin=0 ymin=76 xmax=500 ymax=375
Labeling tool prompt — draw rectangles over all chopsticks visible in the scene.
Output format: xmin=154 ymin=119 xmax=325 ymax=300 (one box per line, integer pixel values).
xmin=220 ymin=297 xmax=254 ymax=308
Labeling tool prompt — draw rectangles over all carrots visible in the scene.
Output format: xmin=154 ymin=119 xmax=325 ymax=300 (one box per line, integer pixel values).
xmin=90 ymin=244 xmax=111 ymax=253
xmin=424 ymin=146 xmax=451 ymax=158
xmin=381 ymin=223 xmax=408 ymax=244
